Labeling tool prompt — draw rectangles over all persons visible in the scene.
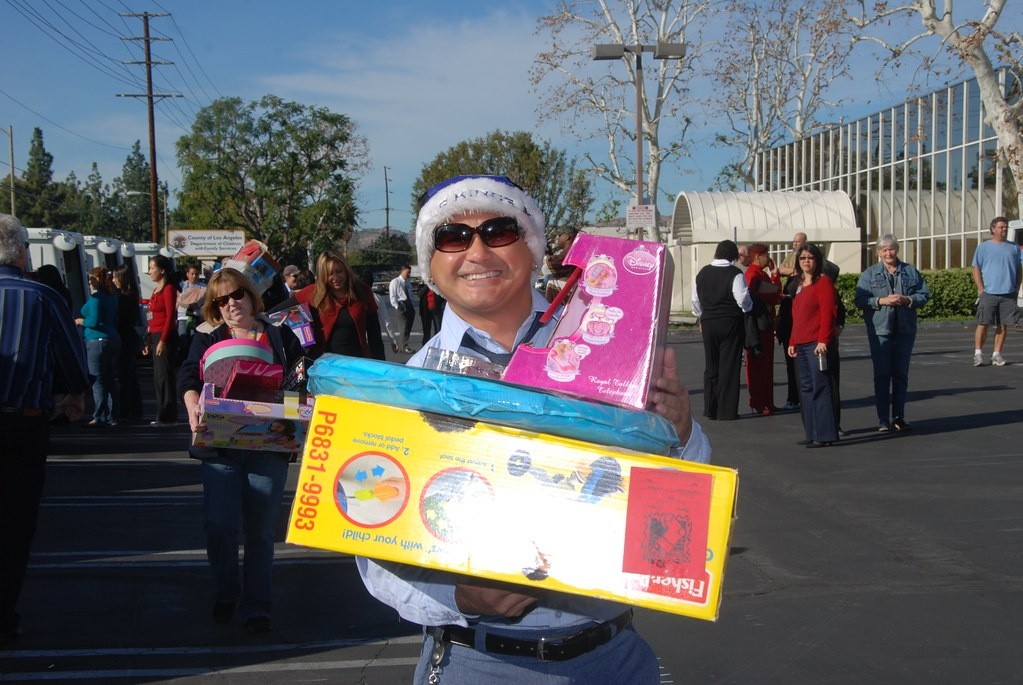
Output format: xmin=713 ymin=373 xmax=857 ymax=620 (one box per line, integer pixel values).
xmin=25 ymin=239 xmax=387 ymax=426
xmin=177 ymin=268 xmax=305 ymax=633
xmin=972 ymin=216 xmax=1023 ymax=367
xmin=535 ymin=226 xmax=586 ymax=307
xmin=354 ymin=174 xmax=711 ymax=685
xmin=691 ymin=232 xmax=850 ymax=448
xmin=854 ymin=233 xmax=932 ymax=432
xmin=1 ymin=211 xmax=91 ymax=639
xmin=389 ymin=262 xmax=446 ymax=354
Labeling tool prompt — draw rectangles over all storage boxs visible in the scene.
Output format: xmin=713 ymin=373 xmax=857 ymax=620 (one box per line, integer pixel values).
xmin=191 ymin=230 xmax=742 ymax=626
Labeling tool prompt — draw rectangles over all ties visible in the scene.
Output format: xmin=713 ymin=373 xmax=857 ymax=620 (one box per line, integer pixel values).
xmin=404 ymin=282 xmax=410 ymax=305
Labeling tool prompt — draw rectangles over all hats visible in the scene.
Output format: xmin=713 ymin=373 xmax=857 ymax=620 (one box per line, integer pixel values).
xmin=283 ymin=265 xmax=301 ymax=275
xmin=415 ymin=175 xmax=545 ymax=297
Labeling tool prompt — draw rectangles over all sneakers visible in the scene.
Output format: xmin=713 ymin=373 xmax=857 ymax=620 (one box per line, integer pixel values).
xmin=992 ymin=355 xmax=1007 ymax=366
xmin=974 ymin=353 xmax=984 ymax=366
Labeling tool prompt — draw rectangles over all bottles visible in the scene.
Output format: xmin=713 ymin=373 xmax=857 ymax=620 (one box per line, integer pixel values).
xmin=818 ymin=352 xmax=827 ymax=371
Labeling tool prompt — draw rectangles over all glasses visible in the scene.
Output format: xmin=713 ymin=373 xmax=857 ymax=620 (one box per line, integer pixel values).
xmin=211 ymin=286 xmax=245 ymax=307
xmin=799 ymin=256 xmax=814 ymax=260
xmin=434 ymin=216 xmax=519 ymax=252
xmin=21 ymin=242 xmax=30 ymax=249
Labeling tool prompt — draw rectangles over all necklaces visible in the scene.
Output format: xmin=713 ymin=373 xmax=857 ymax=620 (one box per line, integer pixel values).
xmin=229 ymin=316 xmax=259 ymax=340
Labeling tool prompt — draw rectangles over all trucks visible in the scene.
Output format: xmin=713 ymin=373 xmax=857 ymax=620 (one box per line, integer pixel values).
xmin=22 ymin=228 xmax=163 ymax=305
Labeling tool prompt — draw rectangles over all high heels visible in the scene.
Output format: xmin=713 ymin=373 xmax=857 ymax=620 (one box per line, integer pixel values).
xmin=751 ymin=404 xmax=782 ymax=414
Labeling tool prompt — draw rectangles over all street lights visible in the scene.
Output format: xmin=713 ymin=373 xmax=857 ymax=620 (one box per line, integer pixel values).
xmin=593 ymin=39 xmax=690 ymax=240
xmin=125 ymin=190 xmax=171 ymax=246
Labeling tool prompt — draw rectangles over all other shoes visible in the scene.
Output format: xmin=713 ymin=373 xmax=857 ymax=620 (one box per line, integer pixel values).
xmin=878 ymin=419 xmax=905 ymax=432
xmin=151 ymin=420 xmax=177 ymax=427
xmin=784 ymin=402 xmax=800 ymax=410
xmin=212 ymin=599 xmax=235 ymax=623
xmin=249 ymin=611 xmax=271 ymax=632
xmin=87 ymin=418 xmax=116 ymax=427
xmin=400 ymin=348 xmax=415 ymax=354
xmin=798 ymin=439 xmax=833 ymax=449
xmin=708 ymin=413 xmax=740 ymax=420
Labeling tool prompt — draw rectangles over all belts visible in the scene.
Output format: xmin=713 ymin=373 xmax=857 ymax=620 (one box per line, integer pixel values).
xmin=426 ymin=608 xmax=634 ymax=663
